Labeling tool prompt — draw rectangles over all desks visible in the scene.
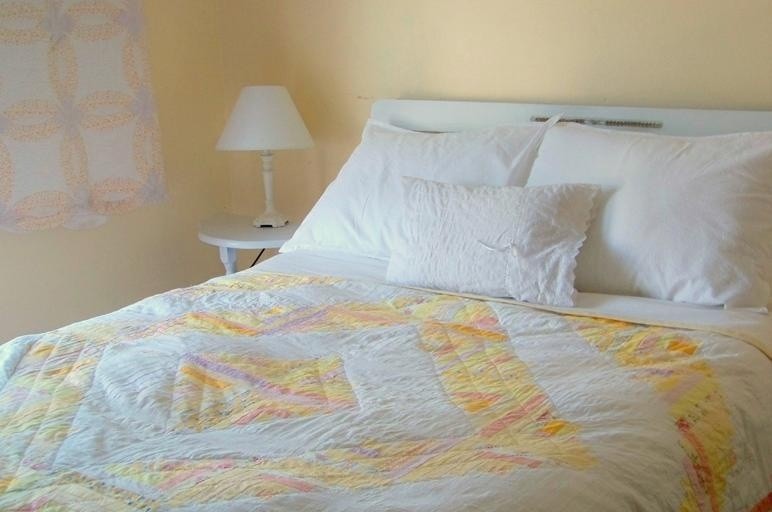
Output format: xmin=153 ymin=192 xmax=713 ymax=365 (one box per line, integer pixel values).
xmin=194 ymin=217 xmax=296 ymax=274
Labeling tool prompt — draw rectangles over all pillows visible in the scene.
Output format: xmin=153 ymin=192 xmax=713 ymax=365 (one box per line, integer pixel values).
xmin=382 ymin=174 xmax=604 ymax=309
xmin=275 ymin=114 xmax=562 ymax=262
xmin=523 ymin=122 xmax=772 ymax=308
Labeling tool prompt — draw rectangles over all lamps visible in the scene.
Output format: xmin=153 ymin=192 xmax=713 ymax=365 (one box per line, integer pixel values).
xmin=214 ymin=83 xmax=316 ymax=228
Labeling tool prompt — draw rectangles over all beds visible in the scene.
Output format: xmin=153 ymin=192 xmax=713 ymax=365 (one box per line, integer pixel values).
xmin=0 ymin=94 xmax=771 ymax=512
